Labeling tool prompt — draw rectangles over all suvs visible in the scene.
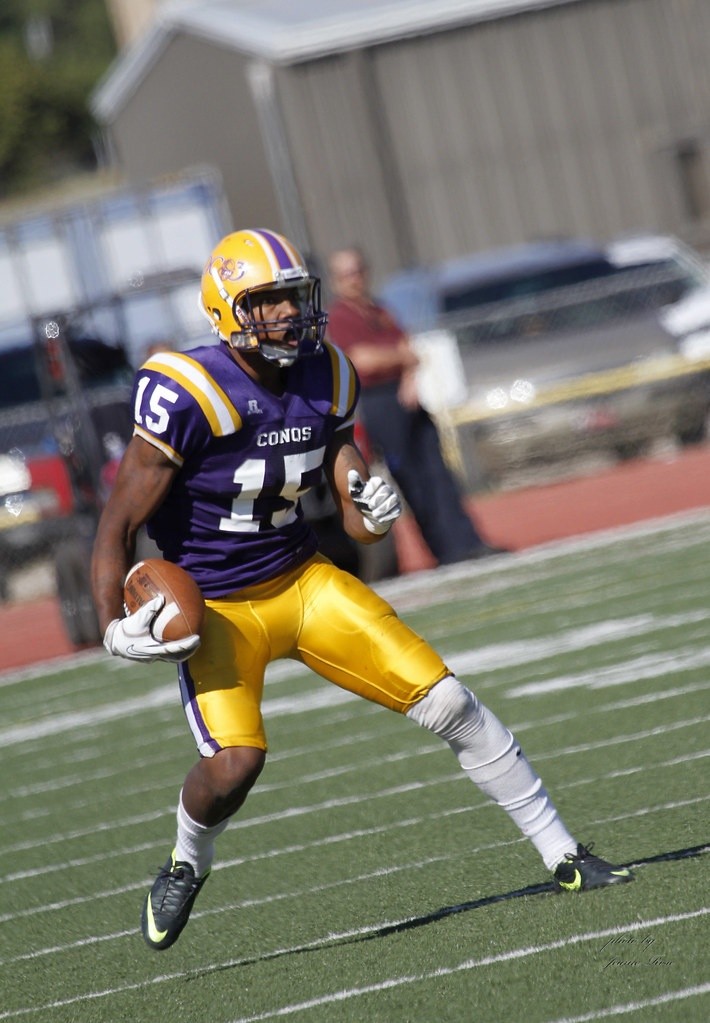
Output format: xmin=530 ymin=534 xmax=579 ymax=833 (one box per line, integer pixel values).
xmin=1 ymin=330 xmax=149 ymax=592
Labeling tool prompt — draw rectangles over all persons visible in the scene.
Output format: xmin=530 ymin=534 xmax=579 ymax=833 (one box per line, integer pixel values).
xmin=323 ymin=242 xmax=513 ymax=567
xmin=91 ymin=229 xmax=634 ymax=950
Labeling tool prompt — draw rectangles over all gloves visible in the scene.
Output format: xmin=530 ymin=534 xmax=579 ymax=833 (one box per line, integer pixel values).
xmin=347 ymin=468 xmax=400 ymax=535
xmin=103 ymin=597 xmax=202 ymax=664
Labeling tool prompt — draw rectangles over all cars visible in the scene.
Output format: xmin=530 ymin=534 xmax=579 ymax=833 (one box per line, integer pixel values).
xmin=384 ymin=237 xmax=709 ymax=489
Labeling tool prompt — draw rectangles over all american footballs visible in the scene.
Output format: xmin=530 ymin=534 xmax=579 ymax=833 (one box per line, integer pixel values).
xmin=120 ymin=556 xmax=203 ymax=647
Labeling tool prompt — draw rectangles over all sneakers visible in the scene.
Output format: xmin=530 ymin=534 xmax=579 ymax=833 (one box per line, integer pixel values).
xmin=140 ymin=853 xmax=209 ymax=951
xmin=552 ymin=842 xmax=636 ymax=893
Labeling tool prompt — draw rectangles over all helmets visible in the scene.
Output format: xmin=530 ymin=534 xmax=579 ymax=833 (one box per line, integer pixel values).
xmin=202 ymin=229 xmax=329 ymax=381
